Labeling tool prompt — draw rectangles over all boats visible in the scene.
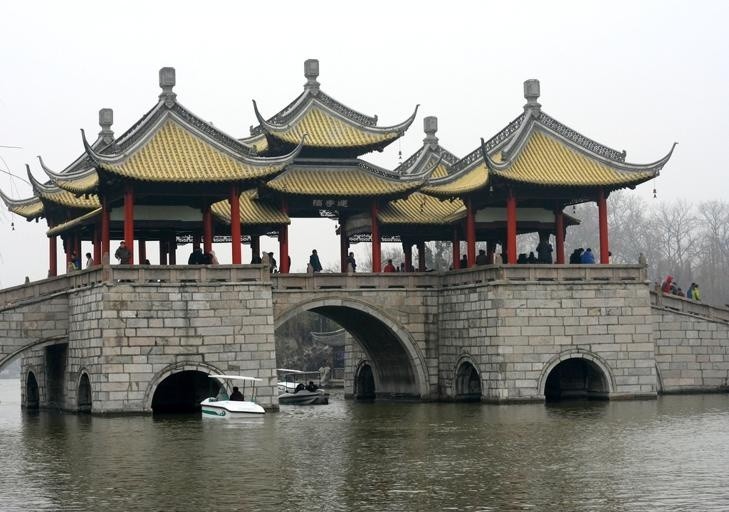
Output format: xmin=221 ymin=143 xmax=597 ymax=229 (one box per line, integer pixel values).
xmin=278 ymin=367 xmax=330 ymax=405
xmin=199 ymin=374 xmax=267 ymax=420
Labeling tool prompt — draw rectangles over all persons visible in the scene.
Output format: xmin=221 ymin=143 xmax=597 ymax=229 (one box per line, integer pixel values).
xmin=215 ymin=386 xmax=229 ymax=400
xmin=295 ymin=384 xmax=304 ymax=393
xmin=306 ymin=381 xmax=319 ymax=393
xmin=230 ymin=387 xmax=245 ymax=401
